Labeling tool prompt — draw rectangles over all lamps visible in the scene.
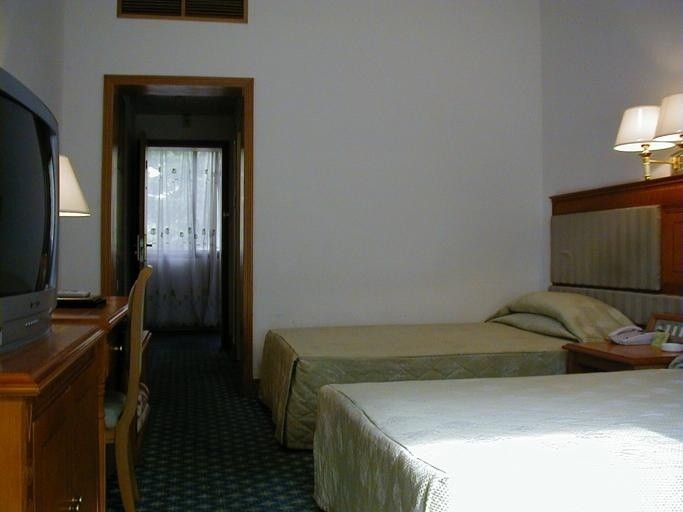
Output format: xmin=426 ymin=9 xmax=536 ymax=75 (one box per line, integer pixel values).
xmin=612 ymin=91 xmax=683 ymax=179
xmin=58 ymin=154 xmax=90 ymax=216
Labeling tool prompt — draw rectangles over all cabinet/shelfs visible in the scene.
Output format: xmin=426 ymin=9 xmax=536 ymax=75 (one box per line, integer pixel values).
xmin=0 ymin=295 xmax=129 ymax=511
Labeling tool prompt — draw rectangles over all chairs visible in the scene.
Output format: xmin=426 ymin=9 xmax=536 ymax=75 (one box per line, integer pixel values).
xmin=103 ymin=264 xmax=153 ymax=511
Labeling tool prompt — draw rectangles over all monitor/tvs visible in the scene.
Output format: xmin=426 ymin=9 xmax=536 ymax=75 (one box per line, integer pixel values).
xmin=0 ymin=67 xmax=60 ymax=354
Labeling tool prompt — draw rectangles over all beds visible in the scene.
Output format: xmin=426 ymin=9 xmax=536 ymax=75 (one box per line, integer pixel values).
xmin=257 ymin=177 xmax=682 ymax=449
xmin=312 ymin=369 xmax=682 ymax=512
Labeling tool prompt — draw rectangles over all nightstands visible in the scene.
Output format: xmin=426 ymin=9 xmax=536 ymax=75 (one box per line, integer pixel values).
xmin=562 ymin=312 xmax=683 ymax=374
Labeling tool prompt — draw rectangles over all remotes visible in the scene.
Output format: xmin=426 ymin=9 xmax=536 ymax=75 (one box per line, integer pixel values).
xmin=56 ymin=288 xmax=91 ymax=298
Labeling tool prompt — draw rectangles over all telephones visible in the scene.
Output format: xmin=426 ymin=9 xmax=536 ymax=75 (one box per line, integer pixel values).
xmin=608 ymin=325 xmax=656 ymax=345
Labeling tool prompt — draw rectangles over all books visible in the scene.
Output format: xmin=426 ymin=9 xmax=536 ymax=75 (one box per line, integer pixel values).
xmin=55 ymin=294 xmax=101 ymax=302
xmin=56 ymin=298 xmax=107 ymax=309
